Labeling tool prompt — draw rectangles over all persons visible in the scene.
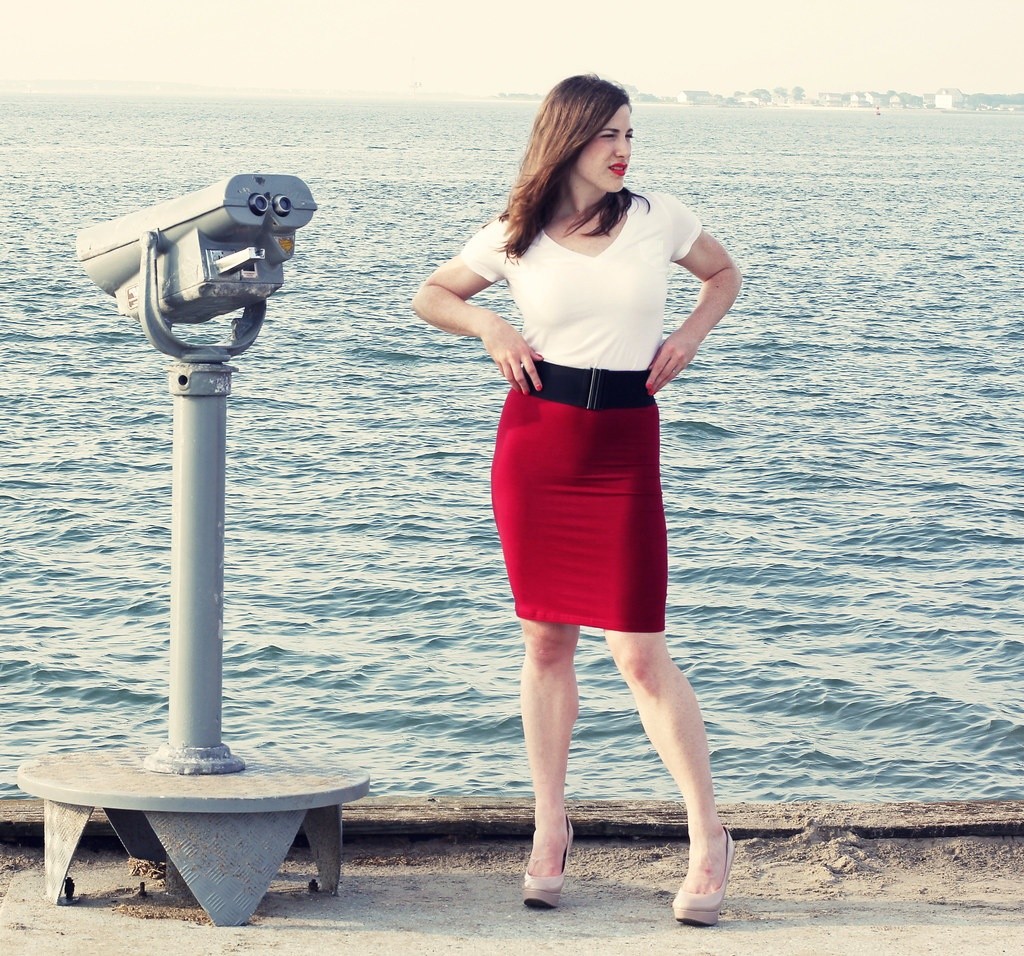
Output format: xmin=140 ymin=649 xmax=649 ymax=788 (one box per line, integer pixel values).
xmin=410 ymin=74 xmax=737 ymax=932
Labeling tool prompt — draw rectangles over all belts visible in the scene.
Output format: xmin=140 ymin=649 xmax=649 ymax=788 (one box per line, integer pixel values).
xmin=520 ymin=360 xmax=656 ymax=412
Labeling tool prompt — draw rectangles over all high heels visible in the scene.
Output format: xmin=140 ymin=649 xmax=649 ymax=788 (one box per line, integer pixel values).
xmin=672 ymin=827 xmax=735 ymax=924
xmin=520 ymin=816 xmax=576 ymax=908
